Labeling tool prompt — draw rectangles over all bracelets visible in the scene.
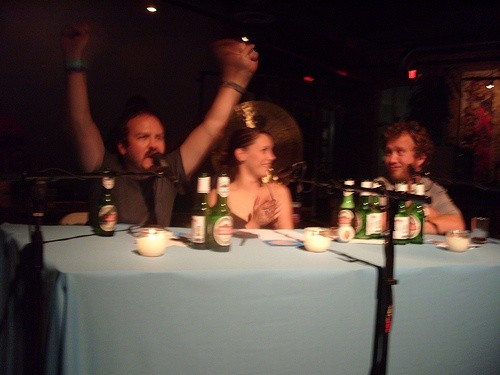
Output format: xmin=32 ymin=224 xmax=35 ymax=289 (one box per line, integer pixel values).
xmin=64 ymin=57 xmax=90 ymax=73
xmin=424 ymin=219 xmax=440 ymax=234
xmin=218 ymin=79 xmax=247 ymax=95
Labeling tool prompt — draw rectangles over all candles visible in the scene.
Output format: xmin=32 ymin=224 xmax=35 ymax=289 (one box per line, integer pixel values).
xmin=137 ymin=227 xmax=168 ymax=257
xmin=305 ymin=223 xmax=333 ymax=254
xmin=445 ymin=228 xmax=469 ymax=251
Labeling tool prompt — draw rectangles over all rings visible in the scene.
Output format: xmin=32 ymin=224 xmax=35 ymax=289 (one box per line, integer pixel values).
xmin=266 ymin=209 xmax=271 ymax=214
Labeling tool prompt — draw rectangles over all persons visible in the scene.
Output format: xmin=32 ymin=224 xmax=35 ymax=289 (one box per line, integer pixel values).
xmin=202 ymin=127 xmax=296 ymax=232
xmin=357 ymin=119 xmax=466 ymax=234
xmin=61 ymin=13 xmax=259 ymax=227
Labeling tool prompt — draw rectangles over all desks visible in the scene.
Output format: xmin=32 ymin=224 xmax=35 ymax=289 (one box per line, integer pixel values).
xmin=0 ymin=222 xmax=500 ymax=375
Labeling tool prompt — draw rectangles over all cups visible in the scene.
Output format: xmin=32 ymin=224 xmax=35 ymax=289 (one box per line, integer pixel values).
xmin=471 ymin=217 xmax=489 ymax=244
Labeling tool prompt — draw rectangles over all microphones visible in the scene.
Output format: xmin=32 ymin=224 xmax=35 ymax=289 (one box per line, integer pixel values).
xmin=154 ymin=152 xmax=181 ymax=185
xmin=272 ymin=162 xmax=291 ymax=184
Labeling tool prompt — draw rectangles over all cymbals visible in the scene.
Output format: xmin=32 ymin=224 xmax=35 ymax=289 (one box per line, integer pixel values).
xmin=210 ymin=99 xmax=304 ymax=186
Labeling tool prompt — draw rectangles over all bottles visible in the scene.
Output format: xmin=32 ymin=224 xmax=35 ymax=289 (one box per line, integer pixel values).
xmin=190 ymin=174 xmax=212 ymax=250
xmin=337 ymin=179 xmax=425 ymax=245
xmin=206 ymin=173 xmax=234 ymax=252
xmin=95 ymin=168 xmax=118 ymax=238
